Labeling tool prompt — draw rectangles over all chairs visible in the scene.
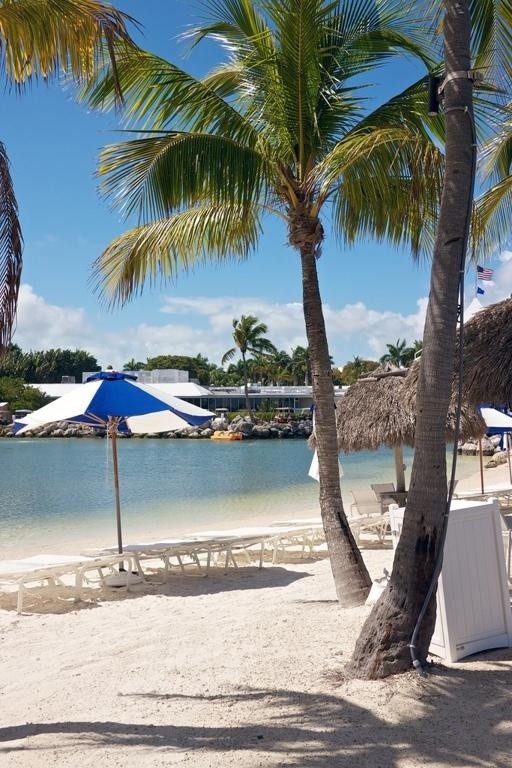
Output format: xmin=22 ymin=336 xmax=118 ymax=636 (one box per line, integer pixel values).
xmin=347 ymin=480 xmax=399 ymax=546
xmin=164 ymin=524 xmax=322 ymax=578
xmin=1 ymin=541 xmax=168 ymax=619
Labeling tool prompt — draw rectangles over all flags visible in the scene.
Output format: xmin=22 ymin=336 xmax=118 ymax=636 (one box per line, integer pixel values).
xmin=477 ymin=266 xmax=493 ymax=281
xmin=477 ymin=288 xmax=484 ymax=294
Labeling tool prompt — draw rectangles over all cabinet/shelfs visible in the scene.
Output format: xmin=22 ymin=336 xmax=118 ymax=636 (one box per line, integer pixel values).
xmin=387 ymin=495 xmax=512 ymax=665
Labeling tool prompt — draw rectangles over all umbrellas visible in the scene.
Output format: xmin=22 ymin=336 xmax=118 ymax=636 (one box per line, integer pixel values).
xmin=307 ymin=402 xmax=344 ymax=481
xmin=477 ymin=403 xmax=512 ymax=494
xmin=12 ymin=365 xmax=218 ymax=568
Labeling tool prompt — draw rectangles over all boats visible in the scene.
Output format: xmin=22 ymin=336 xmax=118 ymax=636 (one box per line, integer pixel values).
xmin=211 ymin=430 xmax=242 ymax=441
